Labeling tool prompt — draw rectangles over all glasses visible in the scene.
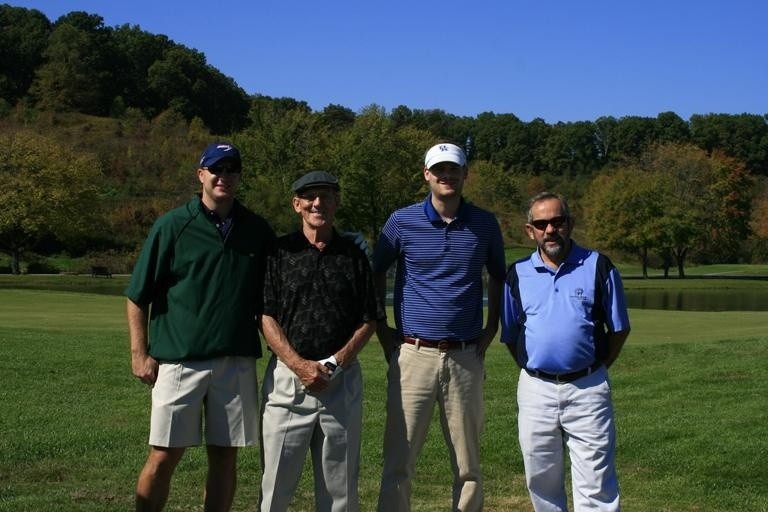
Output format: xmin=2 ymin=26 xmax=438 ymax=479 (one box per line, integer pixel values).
xmin=208 ymin=165 xmax=242 ymax=174
xmin=299 ymin=192 xmax=336 ymax=199
xmin=532 ymin=216 xmax=565 ymax=229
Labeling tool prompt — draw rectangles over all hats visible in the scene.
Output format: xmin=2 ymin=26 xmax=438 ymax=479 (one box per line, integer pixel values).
xmin=425 ymin=143 xmax=466 ymax=170
xmin=291 ymin=170 xmax=339 ymax=192
xmin=200 ymin=142 xmax=241 ymax=166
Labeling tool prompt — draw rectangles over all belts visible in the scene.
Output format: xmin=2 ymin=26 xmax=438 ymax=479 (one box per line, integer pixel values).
xmin=539 ymin=361 xmax=601 ymax=384
xmin=404 ymin=337 xmax=461 ymax=352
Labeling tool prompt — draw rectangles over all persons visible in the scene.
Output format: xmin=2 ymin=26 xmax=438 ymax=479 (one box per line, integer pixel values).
xmin=372 ymin=143 xmax=506 ymax=512
xmin=258 ymin=172 xmax=386 ymax=512
xmin=124 ymin=143 xmax=370 ymax=512
xmin=499 ymin=192 xmax=630 ymax=512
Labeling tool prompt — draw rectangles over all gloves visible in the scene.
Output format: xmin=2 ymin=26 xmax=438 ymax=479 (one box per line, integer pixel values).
xmin=299 ymin=355 xmax=343 ymax=396
